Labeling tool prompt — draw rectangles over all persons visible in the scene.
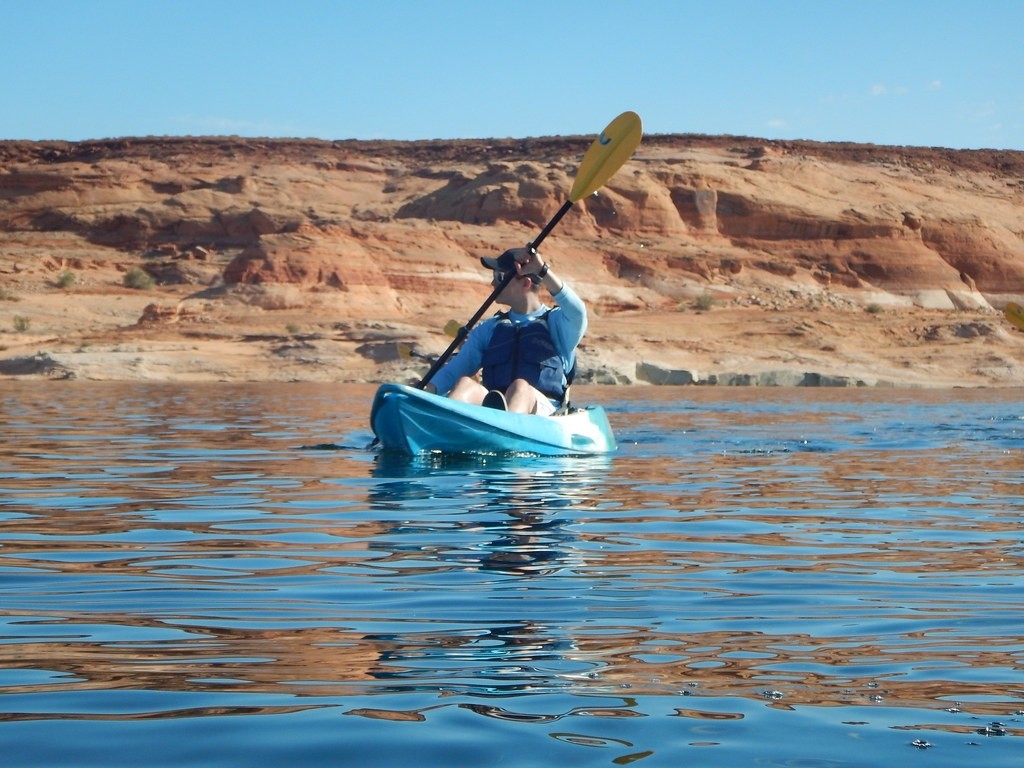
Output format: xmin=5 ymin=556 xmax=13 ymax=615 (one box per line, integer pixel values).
xmin=407 ymin=248 xmax=588 ymax=416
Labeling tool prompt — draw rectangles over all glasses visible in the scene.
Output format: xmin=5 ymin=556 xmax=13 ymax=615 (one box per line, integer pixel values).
xmin=493 ymin=272 xmax=507 ymax=282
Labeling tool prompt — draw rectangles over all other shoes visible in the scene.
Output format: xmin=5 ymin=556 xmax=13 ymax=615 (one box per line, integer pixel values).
xmin=482 ymin=389 xmax=508 ymax=412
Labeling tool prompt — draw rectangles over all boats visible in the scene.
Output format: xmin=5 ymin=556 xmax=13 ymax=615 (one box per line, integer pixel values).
xmin=366 ymin=379 xmax=619 ymax=464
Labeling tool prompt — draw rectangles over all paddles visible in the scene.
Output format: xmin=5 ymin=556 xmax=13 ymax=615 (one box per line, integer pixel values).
xmin=368 ymin=108 xmax=644 ymax=449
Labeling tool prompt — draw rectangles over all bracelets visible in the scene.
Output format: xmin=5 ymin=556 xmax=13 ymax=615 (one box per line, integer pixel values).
xmin=537 ymin=262 xmax=550 ymax=281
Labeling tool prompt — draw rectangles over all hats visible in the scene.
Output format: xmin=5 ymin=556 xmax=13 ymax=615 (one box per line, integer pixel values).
xmin=480 ymin=248 xmax=543 ymax=285
